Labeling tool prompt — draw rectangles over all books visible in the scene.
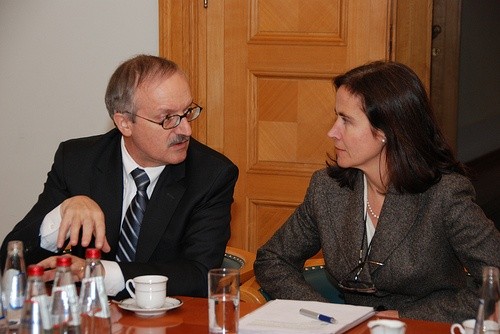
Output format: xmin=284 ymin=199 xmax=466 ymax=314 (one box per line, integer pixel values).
xmin=239 ymin=298 xmax=374 ymax=334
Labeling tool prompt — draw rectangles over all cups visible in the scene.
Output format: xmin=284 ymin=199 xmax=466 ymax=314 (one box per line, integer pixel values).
xmin=208 ymin=268 xmax=240 ymax=334
xmin=125 ymin=275 xmax=169 ymax=310
xmin=450 ymin=319 xmax=500 ymax=334
xmin=368 ymin=318 xmax=407 ymax=334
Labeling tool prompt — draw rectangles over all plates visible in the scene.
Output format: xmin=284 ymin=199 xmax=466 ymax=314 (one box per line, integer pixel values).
xmin=118 ymin=297 xmax=183 ymax=316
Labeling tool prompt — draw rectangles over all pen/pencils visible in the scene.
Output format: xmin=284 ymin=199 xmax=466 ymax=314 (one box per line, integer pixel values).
xmin=300 ymin=308 xmax=335 ymax=324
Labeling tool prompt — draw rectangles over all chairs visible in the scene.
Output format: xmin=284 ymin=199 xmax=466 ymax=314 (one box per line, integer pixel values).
xmin=217 ymin=246 xmax=344 ymax=306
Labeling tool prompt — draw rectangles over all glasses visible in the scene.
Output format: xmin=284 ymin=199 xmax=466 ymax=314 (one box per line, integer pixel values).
xmin=119 ymin=101 xmax=202 ymax=129
xmin=337 ymin=261 xmax=384 ymax=293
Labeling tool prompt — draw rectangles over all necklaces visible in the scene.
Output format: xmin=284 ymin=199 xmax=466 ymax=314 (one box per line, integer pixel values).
xmin=367 ymin=201 xmax=379 ymax=218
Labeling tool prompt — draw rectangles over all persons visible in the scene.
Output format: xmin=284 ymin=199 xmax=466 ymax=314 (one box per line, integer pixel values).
xmin=0 ymin=55 xmax=240 ymax=300
xmin=253 ymin=60 xmax=500 ymax=322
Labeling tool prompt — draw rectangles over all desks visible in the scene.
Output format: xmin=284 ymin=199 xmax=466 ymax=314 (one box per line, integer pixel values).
xmin=108 ymin=294 xmax=463 ymax=334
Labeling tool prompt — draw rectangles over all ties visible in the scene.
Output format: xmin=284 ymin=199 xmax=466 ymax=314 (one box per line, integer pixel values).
xmin=113 ymin=168 xmax=150 ymax=264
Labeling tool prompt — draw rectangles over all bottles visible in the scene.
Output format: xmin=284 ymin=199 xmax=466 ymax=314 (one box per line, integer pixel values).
xmin=48 ymin=257 xmax=83 ymax=334
xmin=473 ymin=265 xmax=500 ymax=334
xmin=78 ymin=248 xmax=112 ymax=334
xmin=19 ymin=264 xmax=54 ymax=334
xmin=0 ymin=241 xmax=26 ymax=334
